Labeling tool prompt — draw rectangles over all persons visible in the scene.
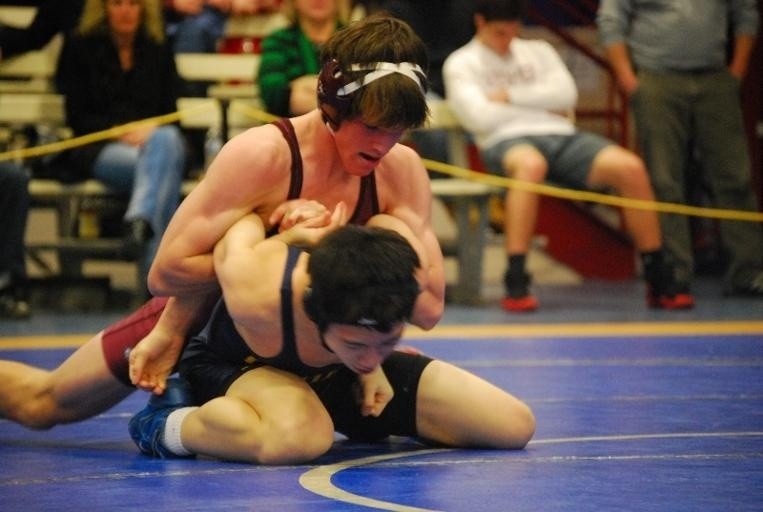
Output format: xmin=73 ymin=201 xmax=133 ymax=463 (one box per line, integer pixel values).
xmin=258 ymin=0 xmax=347 ymax=118
xmin=57 ymin=0 xmax=185 ymax=299
xmin=595 ymin=0 xmax=763 ymax=301
xmin=159 ymin=0 xmax=281 ymax=172
xmin=441 ymin=0 xmax=695 ymax=311
xmin=0 ymin=19 xmax=431 ymax=432
xmin=126 ymin=205 xmax=536 ymax=465
xmin=0 ymin=0 xmax=71 ymax=316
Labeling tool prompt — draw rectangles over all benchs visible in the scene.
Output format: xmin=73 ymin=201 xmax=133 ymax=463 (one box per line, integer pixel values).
xmin=0 ymin=4 xmax=509 ymax=317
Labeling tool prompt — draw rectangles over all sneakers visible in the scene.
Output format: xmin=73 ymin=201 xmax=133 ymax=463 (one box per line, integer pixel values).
xmin=647 ymin=282 xmax=694 ymax=308
xmin=502 ymin=272 xmax=539 ymax=311
xmin=122 ymin=219 xmax=149 ymax=259
xmin=731 ymin=266 xmax=762 ymax=297
xmin=1 ymin=291 xmax=27 ymax=317
xmin=127 ymin=376 xmax=199 ymax=459
